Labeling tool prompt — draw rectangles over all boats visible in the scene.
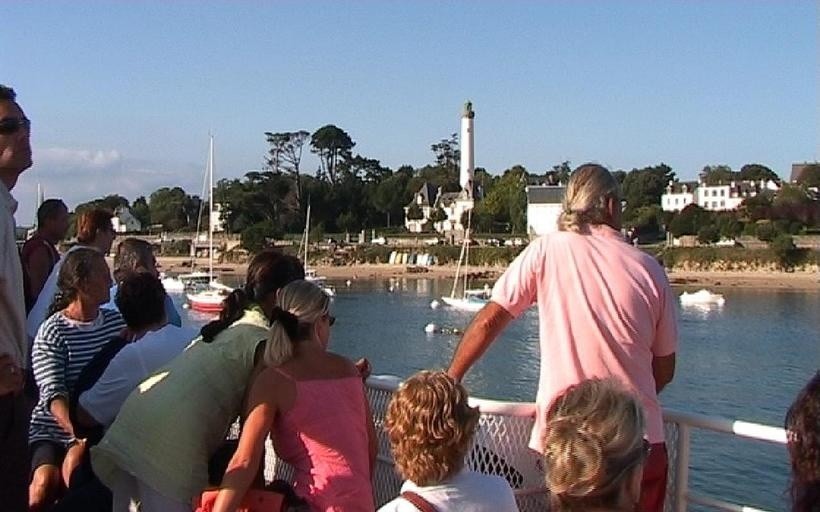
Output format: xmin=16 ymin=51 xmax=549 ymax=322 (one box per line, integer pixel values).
xmin=678 ymin=287 xmax=726 ymax=304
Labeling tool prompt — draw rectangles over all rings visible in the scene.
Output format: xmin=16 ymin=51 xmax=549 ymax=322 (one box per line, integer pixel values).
xmin=10 ymin=365 xmax=15 ymax=375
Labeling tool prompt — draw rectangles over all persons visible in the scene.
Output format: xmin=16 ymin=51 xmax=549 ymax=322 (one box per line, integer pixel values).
xmin=783 ymin=368 xmax=819 ymax=512
xmin=0 ymin=84 xmax=34 ymax=443
xmin=90 ymin=251 xmax=378 ymax=511
xmin=21 ymin=199 xmax=199 ymax=511
xmin=445 ymin=164 xmax=679 ymax=511
xmin=539 ymin=375 xmax=654 ymax=511
xmin=372 ymin=367 xmax=521 ymax=511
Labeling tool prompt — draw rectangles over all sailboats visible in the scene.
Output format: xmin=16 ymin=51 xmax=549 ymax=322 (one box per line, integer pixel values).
xmin=159 ymin=120 xmax=236 ymax=314
xmin=297 ymin=185 xmax=327 ymax=286
xmin=442 ymin=181 xmax=493 ymax=312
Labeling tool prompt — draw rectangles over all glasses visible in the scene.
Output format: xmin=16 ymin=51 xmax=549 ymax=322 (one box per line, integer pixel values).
xmin=323 ymin=313 xmax=336 ymax=326
xmin=616 ymin=200 xmax=628 ymax=211
xmin=0 ymin=116 xmax=30 ymax=135
